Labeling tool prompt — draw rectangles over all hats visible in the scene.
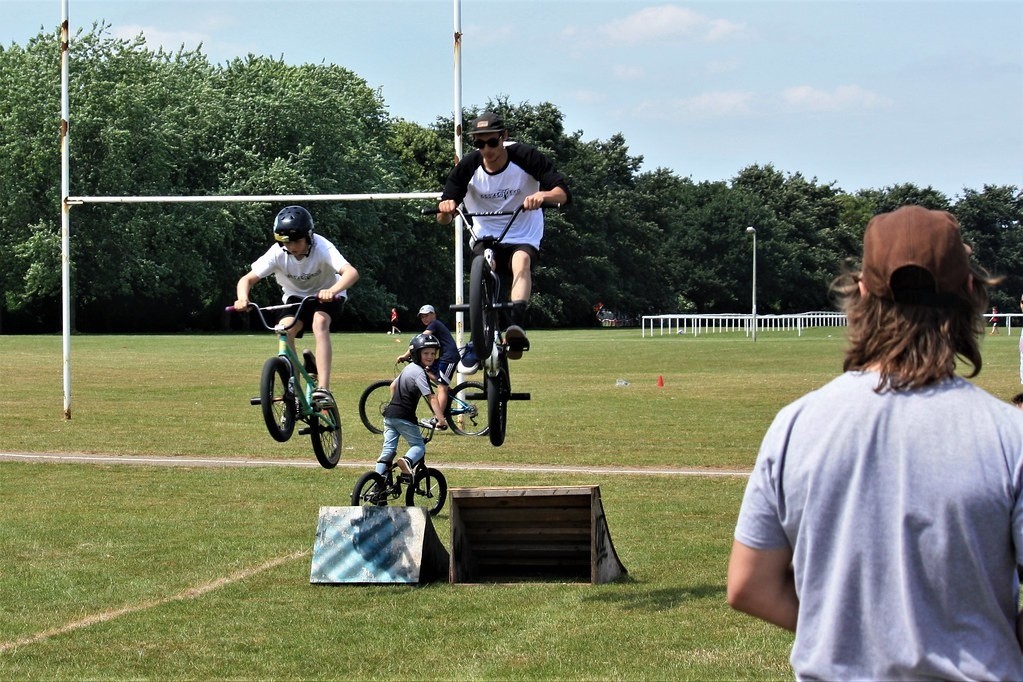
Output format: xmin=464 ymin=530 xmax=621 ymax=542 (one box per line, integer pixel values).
xmin=466 ymin=111 xmax=505 ymax=133
xmin=863 ymin=205 xmax=970 ymax=300
xmin=417 ymin=305 xmax=435 ymax=317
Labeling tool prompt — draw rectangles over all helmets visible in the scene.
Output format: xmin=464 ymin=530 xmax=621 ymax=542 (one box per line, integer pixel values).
xmin=273 ymin=206 xmax=314 ymax=247
xmin=409 ymin=333 xmax=440 ymax=365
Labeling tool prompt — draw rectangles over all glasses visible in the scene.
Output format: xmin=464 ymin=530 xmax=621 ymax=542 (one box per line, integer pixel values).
xmin=473 ymin=132 xmax=504 ymax=150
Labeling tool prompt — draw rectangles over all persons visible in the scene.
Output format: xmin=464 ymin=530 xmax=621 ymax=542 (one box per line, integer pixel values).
xmin=375 ymin=334 xmax=448 ymax=504
xmin=726 ymin=205 xmax=1023 ymax=682
xmin=989 ymin=306 xmax=1000 ymax=335
xmin=396 ymin=305 xmax=461 ymax=431
xmin=439 ymin=111 xmax=572 ymax=375
xmin=234 ymin=206 xmax=359 ymax=410
xmin=391 ymin=308 xmax=401 ymax=335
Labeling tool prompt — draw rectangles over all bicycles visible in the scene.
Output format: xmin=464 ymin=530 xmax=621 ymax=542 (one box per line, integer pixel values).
xmin=350 ymin=418 xmax=448 ymax=517
xmin=358 ymin=359 xmax=490 ymax=438
xmin=225 ymin=295 xmax=343 ymax=470
xmin=419 ymin=203 xmax=559 ymax=447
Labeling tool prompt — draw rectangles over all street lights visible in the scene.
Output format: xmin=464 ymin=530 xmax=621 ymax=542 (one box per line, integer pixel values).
xmin=747 ymin=226 xmax=757 ymax=342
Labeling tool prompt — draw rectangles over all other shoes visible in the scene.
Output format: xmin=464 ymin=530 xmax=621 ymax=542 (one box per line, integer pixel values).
xmin=280 ymin=397 xmax=301 ymax=424
xmin=370 ymin=491 xmax=388 ymax=507
xmin=397 ymin=458 xmax=415 ymax=485
xmin=421 ymin=417 xmax=441 ymax=431
xmin=456 ymin=341 xmax=479 ymax=375
xmin=504 ymin=325 xmax=525 ymax=360
xmin=310 ymin=388 xmax=335 ymax=410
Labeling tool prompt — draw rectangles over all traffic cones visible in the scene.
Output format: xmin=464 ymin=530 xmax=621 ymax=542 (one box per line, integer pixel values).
xmin=658 ymin=374 xmax=665 ymax=387
